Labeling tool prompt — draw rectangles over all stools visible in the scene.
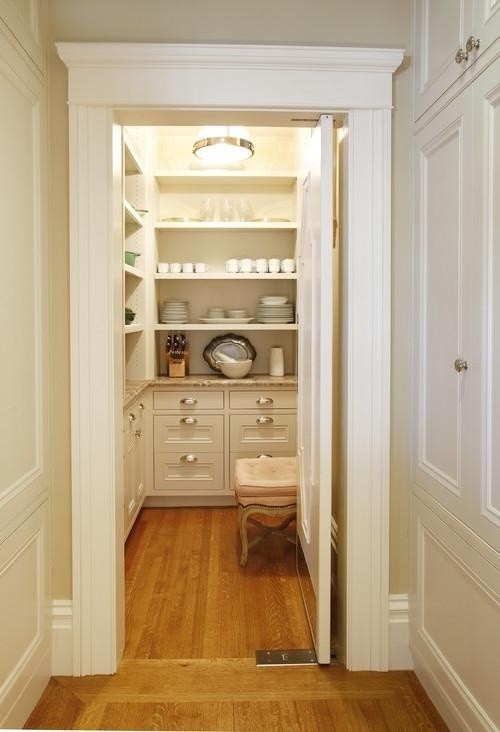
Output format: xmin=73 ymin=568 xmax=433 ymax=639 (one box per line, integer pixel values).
xmin=234 ymin=456 xmax=298 ymax=567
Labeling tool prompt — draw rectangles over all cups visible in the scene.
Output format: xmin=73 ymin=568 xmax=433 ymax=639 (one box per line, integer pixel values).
xmin=238 ymin=199 xmax=254 ymax=222
xmin=219 ymin=198 xmax=234 ymax=222
xmin=199 ymin=197 xmax=215 ymax=222
xmin=158 ymin=258 xmax=295 ymax=273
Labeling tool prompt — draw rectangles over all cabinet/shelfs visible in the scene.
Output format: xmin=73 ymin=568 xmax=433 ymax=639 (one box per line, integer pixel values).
xmin=122 ymin=127 xmax=299 ymax=380
xmin=122 ymin=381 xmax=151 ymax=543
xmin=0 ymin=0 xmax=52 ymax=730
xmin=390 ymin=0 xmax=500 ymax=731
xmin=144 ymin=381 xmax=298 ymax=508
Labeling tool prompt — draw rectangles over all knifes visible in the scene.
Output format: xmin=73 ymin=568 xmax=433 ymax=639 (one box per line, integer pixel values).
xmin=165 ymin=333 xmax=187 ymax=360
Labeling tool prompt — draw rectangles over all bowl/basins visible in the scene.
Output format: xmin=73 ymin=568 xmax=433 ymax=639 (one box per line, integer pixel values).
xmin=215 ymin=359 xmax=253 ymax=379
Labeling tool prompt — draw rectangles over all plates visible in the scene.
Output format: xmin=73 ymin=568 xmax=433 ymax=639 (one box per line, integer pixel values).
xmin=160 ymin=296 xmax=294 ymax=324
xmin=248 ymin=217 xmax=291 ymax=222
xmin=202 ymin=333 xmax=257 ymax=372
xmin=161 ymin=217 xmax=203 ymax=222
